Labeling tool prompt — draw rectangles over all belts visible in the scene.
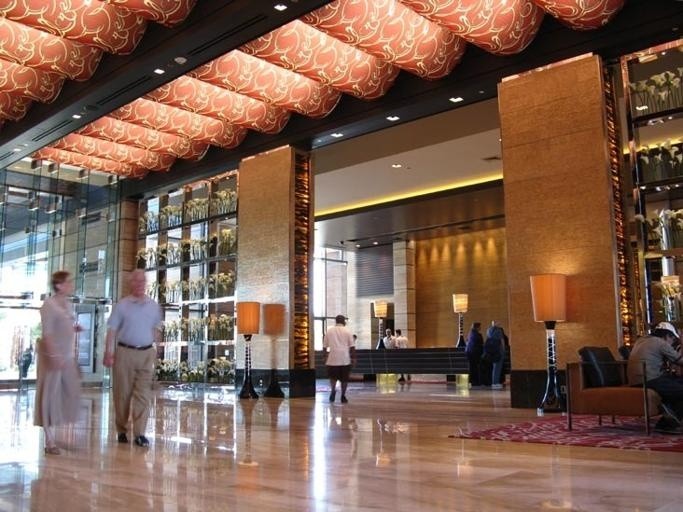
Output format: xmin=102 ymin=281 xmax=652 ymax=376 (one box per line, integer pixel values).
xmin=116 ymin=341 xmax=154 ymax=351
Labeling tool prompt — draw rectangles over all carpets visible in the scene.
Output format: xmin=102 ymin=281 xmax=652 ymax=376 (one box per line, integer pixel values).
xmin=447 ymin=416 xmax=683 ymax=453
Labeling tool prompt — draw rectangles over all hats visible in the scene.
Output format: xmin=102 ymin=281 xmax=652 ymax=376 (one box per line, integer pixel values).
xmin=335 ymin=314 xmax=348 ymax=320
xmin=655 ymin=321 xmax=680 ymax=339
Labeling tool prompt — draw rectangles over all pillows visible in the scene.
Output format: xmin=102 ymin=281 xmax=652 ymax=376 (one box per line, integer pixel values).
xmin=618 ymin=345 xmax=633 ymax=384
xmin=577 ymin=346 xmax=622 ymax=387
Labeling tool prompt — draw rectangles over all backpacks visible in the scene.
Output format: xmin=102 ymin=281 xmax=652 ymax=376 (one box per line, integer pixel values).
xmin=482 ymin=337 xmax=502 ymax=363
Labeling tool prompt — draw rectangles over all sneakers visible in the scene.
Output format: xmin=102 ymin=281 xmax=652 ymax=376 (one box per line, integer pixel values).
xmin=470 ymin=383 xmax=504 ymax=390
xmin=657 ymin=402 xmax=681 ymax=428
xmin=398 ymin=374 xmax=412 ymax=383
xmin=117 ymin=432 xmax=129 ymax=443
xmin=329 ymin=390 xmax=337 ymax=402
xmin=135 ymin=434 xmax=149 ymax=448
xmin=340 ymin=395 xmax=349 ymax=403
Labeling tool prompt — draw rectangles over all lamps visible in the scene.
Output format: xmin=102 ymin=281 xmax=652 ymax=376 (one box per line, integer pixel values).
xmin=235 ymin=301 xmax=260 ymax=399
xmin=452 ymin=293 xmax=468 ymax=349
xmin=529 ymin=273 xmax=567 ymax=413
xmin=373 ymin=299 xmax=387 ymax=349
xmin=261 ymin=303 xmax=286 ymax=398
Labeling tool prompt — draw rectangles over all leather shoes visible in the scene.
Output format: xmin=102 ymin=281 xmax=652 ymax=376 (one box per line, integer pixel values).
xmin=45 ymin=444 xmax=60 ymax=455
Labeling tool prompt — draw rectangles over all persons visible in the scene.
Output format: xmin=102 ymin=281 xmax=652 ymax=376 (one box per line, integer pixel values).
xmin=383 ymin=328 xmax=395 ymax=350
xmin=100 ymin=267 xmax=163 ymax=447
xmin=31 ymin=270 xmax=84 ymax=455
xmin=482 ymin=319 xmax=507 ymax=390
xmin=464 ymin=322 xmax=484 ymax=391
xmin=323 ymin=315 xmax=356 ymax=405
xmin=321 ymin=409 xmax=358 ymax=500
xmin=624 ymin=321 xmax=683 ymax=432
xmin=395 ymin=330 xmax=412 ymax=383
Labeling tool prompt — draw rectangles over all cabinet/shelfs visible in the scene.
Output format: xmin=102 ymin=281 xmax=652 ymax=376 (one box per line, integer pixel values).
xmin=622 ymin=38 xmax=683 ymax=375
xmin=135 ymin=165 xmax=237 ymax=384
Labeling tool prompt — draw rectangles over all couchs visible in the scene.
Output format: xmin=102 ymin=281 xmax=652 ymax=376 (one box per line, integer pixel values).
xmin=565 ymin=362 xmax=660 ymax=434
